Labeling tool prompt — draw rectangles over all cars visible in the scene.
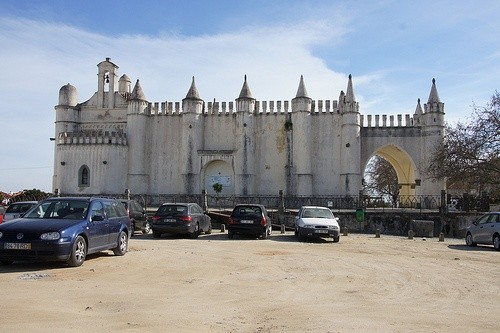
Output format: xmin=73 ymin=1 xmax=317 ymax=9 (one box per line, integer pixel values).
xmin=148 ymin=203 xmax=212 ymax=239
xmin=2 ymin=201 xmax=59 ymax=222
xmin=0 ymin=196 xmax=133 ymax=266
xmin=465 ymin=211 xmax=500 ymax=250
xmin=227 ymin=203 xmax=273 ymax=239
xmin=116 ymin=198 xmax=150 ymax=236
xmin=294 ymin=205 xmax=341 ymax=242
